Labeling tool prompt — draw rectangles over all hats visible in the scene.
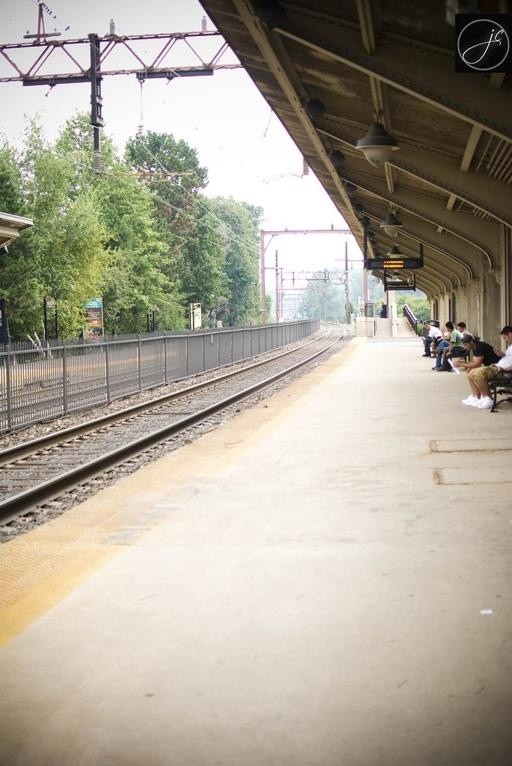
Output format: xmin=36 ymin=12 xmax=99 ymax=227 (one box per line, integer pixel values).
xmin=463 ymin=334 xmax=476 ymax=342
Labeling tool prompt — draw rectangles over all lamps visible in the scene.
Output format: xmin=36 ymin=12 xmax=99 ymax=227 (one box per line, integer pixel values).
xmin=355 ymin=110 xmax=401 ymax=168
xmin=386 ymin=237 xmax=404 ymax=258
xmin=379 ymin=204 xmax=404 ymax=237
xmin=390 ymin=272 xmax=399 ymax=278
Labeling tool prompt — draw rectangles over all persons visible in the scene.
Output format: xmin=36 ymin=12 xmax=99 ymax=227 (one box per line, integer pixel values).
xmin=214 ymin=309 xmax=224 ymax=328
xmin=381 ymin=301 xmax=387 ymax=318
xmin=472 ymin=325 xmax=512 ymax=408
xmin=454 ymin=334 xmax=502 ymax=406
xmin=424 ymin=320 xmax=476 ymax=373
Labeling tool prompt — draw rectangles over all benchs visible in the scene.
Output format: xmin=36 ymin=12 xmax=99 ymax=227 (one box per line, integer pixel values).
xmin=450 ymin=336 xmax=480 ymax=362
xmin=488 ymin=381 xmax=512 ymax=412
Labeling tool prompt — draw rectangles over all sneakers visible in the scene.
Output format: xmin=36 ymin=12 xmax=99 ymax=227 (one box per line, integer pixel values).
xmin=433 ymin=366 xmax=439 ymax=369
xmin=438 ymin=366 xmax=449 ymax=370
xmin=462 ymin=393 xmax=480 ymax=404
xmin=473 ymin=396 xmax=494 ymax=408
